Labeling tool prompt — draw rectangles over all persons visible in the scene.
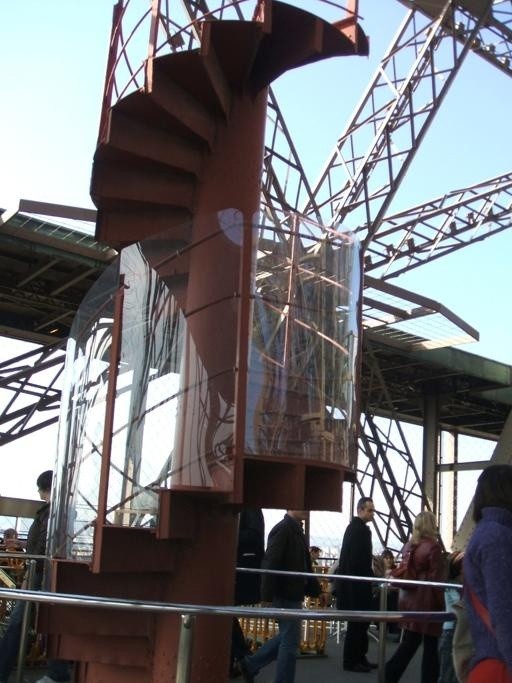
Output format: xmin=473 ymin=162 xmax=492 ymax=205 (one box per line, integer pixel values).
xmin=328 ymin=495 xmax=379 ymax=674
xmin=229 ymin=505 xmax=265 ymax=679
xmin=307 ymin=545 xmax=464 ymax=683
xmin=461 ymin=463 xmax=511 ymax=681
xmin=1 ymin=527 xmax=29 ymax=586
xmin=236 ymin=508 xmax=329 ymax=682
xmin=0 ymin=470 xmax=78 ymax=683
xmin=376 ymin=511 xmax=451 ymax=682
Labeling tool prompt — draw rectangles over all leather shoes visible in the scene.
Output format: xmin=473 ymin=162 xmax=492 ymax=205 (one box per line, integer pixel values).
xmin=342 ymin=655 xmax=379 ymax=673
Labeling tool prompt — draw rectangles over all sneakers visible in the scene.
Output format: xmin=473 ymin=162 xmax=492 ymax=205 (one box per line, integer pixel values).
xmin=239 ymin=659 xmax=258 ymax=683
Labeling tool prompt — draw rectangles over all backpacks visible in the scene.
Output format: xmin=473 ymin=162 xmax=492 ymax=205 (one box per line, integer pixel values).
xmin=390 ymin=542 xmax=419 ymax=590
